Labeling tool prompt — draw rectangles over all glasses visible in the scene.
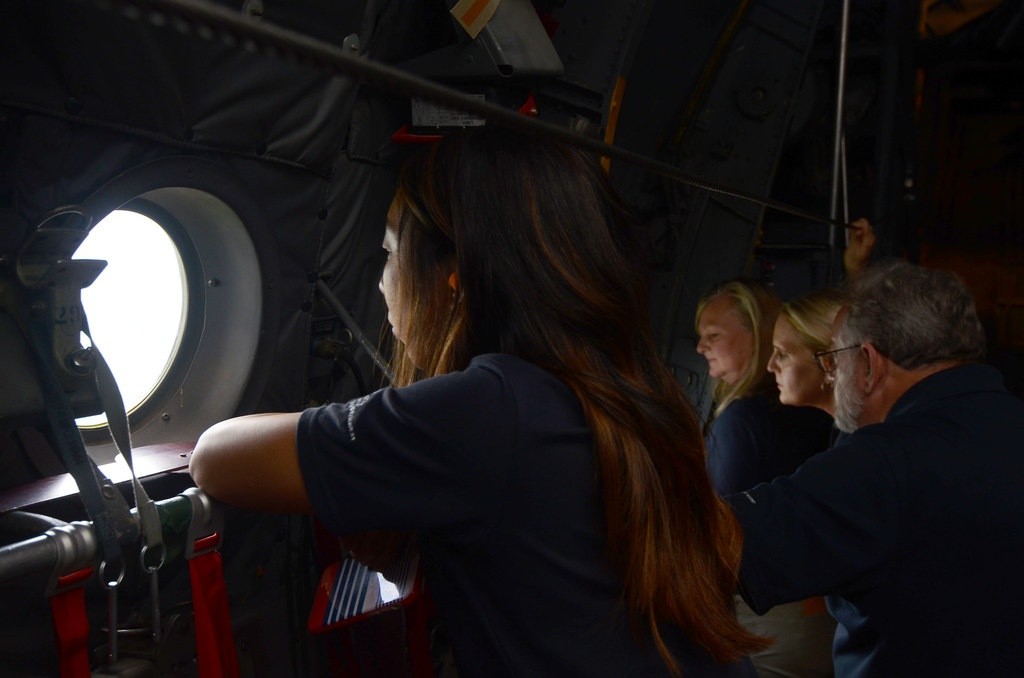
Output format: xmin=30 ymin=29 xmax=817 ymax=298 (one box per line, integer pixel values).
xmin=814 ymin=342 xmax=889 ymax=373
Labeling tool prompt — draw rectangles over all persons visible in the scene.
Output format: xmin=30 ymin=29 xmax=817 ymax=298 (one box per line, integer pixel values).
xmin=694 ymin=279 xmax=837 ymax=678
xmin=189 ymin=126 xmax=776 ymax=678
xmin=722 ymin=263 xmax=1024 ymax=678
xmin=769 ymin=220 xmax=876 ymax=446
